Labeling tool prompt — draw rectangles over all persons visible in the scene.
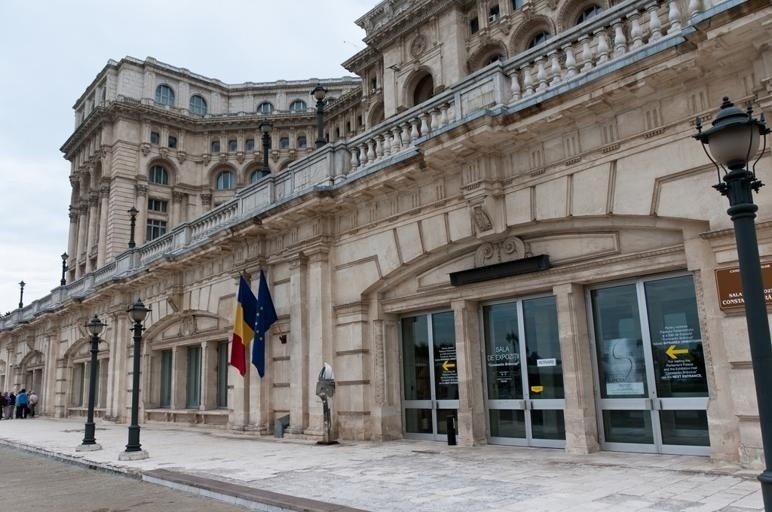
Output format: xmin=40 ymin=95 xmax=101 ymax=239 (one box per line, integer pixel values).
xmin=0 ymin=387 xmax=38 ymax=421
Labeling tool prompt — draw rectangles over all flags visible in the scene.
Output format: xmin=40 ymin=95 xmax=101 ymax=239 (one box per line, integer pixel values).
xmin=226 ymin=275 xmax=258 ymax=378
xmin=248 ymin=270 xmax=279 ymax=379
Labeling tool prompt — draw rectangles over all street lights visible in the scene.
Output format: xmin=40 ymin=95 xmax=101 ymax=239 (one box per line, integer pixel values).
xmin=74 ymin=297 xmax=152 ymax=461
xmin=18 ymin=280 xmax=26 ymax=309
xmin=59 ymin=252 xmax=69 ymax=287
xmin=126 ymin=206 xmax=139 ymax=249
xmin=693 ymin=96 xmax=772 ymax=511
